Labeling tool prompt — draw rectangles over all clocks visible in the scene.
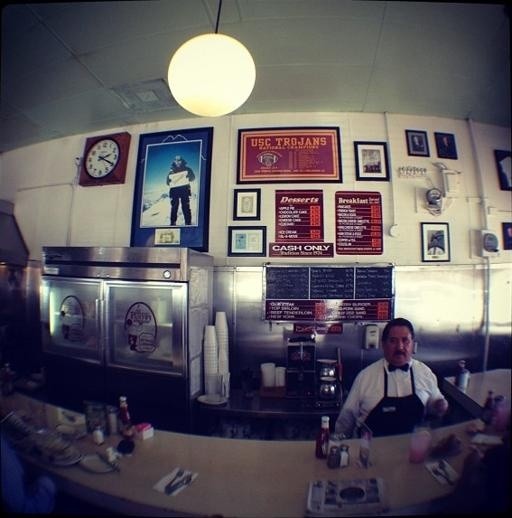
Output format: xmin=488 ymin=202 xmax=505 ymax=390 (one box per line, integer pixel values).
xmin=78 ymin=132 xmax=130 ymax=186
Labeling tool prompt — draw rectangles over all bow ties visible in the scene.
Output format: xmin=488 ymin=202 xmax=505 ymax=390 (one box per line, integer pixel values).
xmin=388 ymin=363 xmax=410 ymax=372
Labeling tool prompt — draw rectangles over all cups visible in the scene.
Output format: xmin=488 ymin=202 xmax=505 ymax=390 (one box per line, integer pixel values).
xmin=203 ymin=310 xmax=229 ymax=374
xmin=261 ymin=363 xmax=275 ymax=387
xmin=205 ymin=373 xmax=221 ymax=402
xmin=275 ymin=367 xmax=285 ymax=386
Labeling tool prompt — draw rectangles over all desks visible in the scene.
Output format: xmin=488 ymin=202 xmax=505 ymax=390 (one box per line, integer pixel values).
xmin=443 ymin=369 xmax=512 ymax=428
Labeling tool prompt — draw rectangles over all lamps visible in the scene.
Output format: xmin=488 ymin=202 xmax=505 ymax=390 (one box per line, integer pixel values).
xmin=167 ymin=0 xmax=256 ymax=118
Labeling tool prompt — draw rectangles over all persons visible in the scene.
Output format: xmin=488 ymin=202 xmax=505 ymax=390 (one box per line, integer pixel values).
xmin=413 ymin=136 xmax=424 ymax=151
xmin=439 ymin=136 xmax=455 ymax=155
xmin=167 ymin=156 xmax=195 ymax=226
xmin=463 ymin=428 xmax=512 ymax=513
xmin=0 ymin=429 xmax=77 ymax=517
xmin=335 ymin=318 xmax=449 ymax=440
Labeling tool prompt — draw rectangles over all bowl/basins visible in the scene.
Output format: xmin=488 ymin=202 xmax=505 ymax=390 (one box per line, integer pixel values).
xmin=55 ymin=448 xmax=72 ymax=459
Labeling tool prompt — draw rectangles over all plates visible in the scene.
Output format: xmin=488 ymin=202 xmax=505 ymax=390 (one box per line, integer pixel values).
xmin=83 ymin=452 xmax=117 ymax=472
xmin=52 ymin=454 xmax=82 ymax=466
xmin=198 ymin=395 xmax=226 ymax=405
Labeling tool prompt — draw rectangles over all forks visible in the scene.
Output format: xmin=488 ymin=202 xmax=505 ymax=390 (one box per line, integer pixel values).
xmin=165 ymin=468 xmax=184 ymax=488
xmin=433 ymin=465 xmax=454 ymax=485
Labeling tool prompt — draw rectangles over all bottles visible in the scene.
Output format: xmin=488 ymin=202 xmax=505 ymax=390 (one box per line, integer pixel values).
xmin=483 ymin=391 xmax=495 ymax=423
xmin=454 ymin=360 xmax=470 ymax=392
xmin=359 ymin=432 xmax=371 ymax=469
xmin=118 ymin=396 xmax=131 ymax=432
xmin=492 ymin=395 xmax=506 ymax=432
xmin=315 ymin=416 xmax=330 ymax=459
xmin=92 ymin=427 xmax=103 ymax=444
xmin=106 ymin=406 xmax=118 ymax=433
xmin=327 ymin=447 xmax=339 ymax=467
xmin=340 ymin=446 xmax=349 ymax=466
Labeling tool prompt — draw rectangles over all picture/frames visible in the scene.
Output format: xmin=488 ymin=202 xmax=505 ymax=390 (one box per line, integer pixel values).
xmin=494 ymin=150 xmax=512 ymax=191
xmin=228 ymin=226 xmax=266 ymax=257
xmin=235 ymin=189 xmax=260 ymax=220
xmin=406 ymin=130 xmax=458 ymax=161
xmin=421 ymin=223 xmax=452 ymax=262
xmin=236 ymin=127 xmax=343 ymax=183
xmin=354 ymin=141 xmax=390 ymax=181
xmin=502 ymin=222 xmax=511 ymax=249
xmin=129 ymin=126 xmax=213 ymax=253
xmin=441 ymin=170 xmax=464 ymax=199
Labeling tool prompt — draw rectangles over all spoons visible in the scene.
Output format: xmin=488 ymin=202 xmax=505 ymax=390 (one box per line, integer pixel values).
xmin=438 ymin=460 xmax=449 ymax=475
xmin=167 ymin=477 xmax=191 ymax=495
